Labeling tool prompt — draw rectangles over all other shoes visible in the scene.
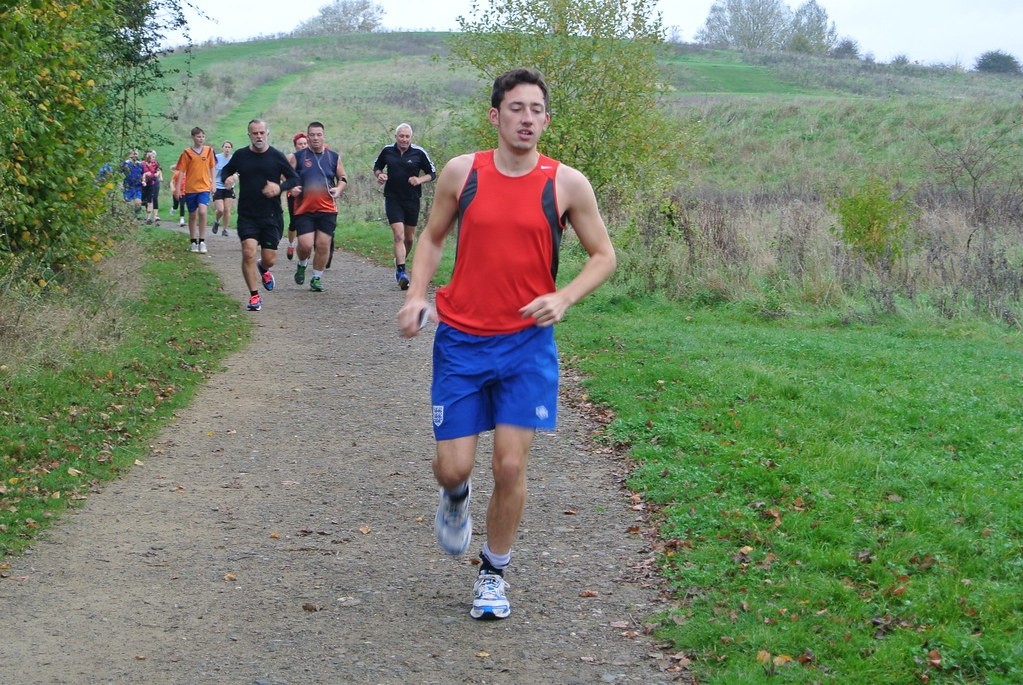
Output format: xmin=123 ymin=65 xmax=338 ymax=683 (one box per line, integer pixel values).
xmin=147 ymin=215 xmax=160 ymax=221
xmin=170 ymin=208 xmax=177 ymax=215
xmin=396 ymin=272 xmax=410 ymax=290
xmin=179 ymin=217 xmax=185 ymax=227
xmin=212 ymin=220 xmax=220 ymax=234
xmin=222 ymin=230 xmax=228 ymax=237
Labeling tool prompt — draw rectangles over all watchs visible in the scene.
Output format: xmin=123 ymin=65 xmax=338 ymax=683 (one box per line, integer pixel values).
xmin=337 ymin=177 xmax=347 ymax=183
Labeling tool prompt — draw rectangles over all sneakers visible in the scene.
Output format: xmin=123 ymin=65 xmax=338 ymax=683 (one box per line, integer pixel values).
xmin=294 ymin=261 xmax=308 ymax=284
xmin=245 ymin=294 xmax=264 ymax=311
xmin=309 ymin=276 xmax=324 ymax=291
xmin=434 ymin=479 xmax=471 ymax=557
xmin=257 ymin=259 xmax=275 ymax=291
xmin=286 ymin=246 xmax=294 ymax=261
xmin=471 ymin=570 xmax=512 ymax=621
xmin=189 ymin=242 xmax=208 ymax=254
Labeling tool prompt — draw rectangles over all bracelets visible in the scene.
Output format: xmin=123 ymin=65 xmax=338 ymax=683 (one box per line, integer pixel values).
xmin=377 ymin=173 xmax=381 ymax=178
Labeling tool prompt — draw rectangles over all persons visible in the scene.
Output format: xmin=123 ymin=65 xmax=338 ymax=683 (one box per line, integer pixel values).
xmin=100 ymin=150 xmax=163 ymax=223
xmin=373 ymin=123 xmax=436 ymax=290
xmin=288 ymin=122 xmax=347 ymax=293
xmin=176 ymin=127 xmax=217 ymax=253
xmin=221 ymin=120 xmax=301 ymax=311
xmin=397 ymin=69 xmax=617 ymax=619
xmin=211 ymin=141 xmax=239 ymax=236
xmin=170 ymin=164 xmax=187 ymax=227
